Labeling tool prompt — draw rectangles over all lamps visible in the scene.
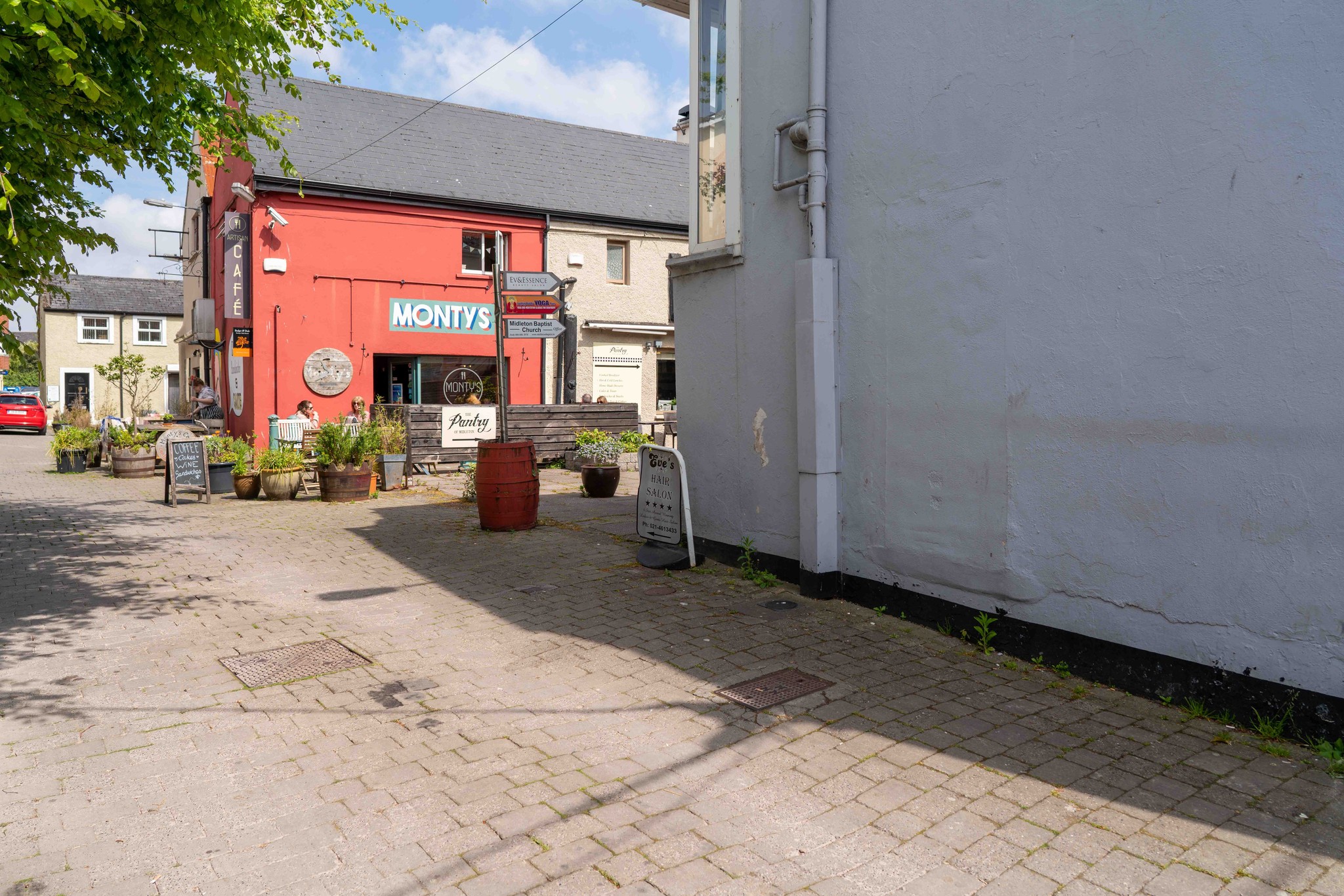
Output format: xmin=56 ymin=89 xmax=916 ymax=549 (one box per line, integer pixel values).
xmin=192 ymin=349 xmax=200 ymax=357
xmin=646 ymin=341 xmax=652 ymax=348
xmin=230 ymin=182 xmax=256 ymax=204
xmin=164 ymin=254 xmax=182 ymax=263
xmin=144 ymin=198 xmax=201 ymax=211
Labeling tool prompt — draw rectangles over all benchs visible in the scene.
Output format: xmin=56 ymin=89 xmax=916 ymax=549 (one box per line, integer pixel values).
xmin=104 ymin=418 xmax=118 ymax=471
xmin=199 ymin=418 xmax=224 ymax=437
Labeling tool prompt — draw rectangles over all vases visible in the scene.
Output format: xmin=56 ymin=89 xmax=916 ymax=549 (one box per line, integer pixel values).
xmin=259 ymin=467 xmax=303 ymax=501
xmin=207 ymin=463 xmax=235 ymax=494
xmin=657 ymin=407 xmax=663 ymax=411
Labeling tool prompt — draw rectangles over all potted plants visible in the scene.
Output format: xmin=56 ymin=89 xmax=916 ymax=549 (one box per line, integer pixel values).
xmin=666 ymin=397 xmax=677 ymax=411
xmin=581 ymin=461 xmax=621 ymax=497
xmin=48 ymin=386 xmax=103 ymax=472
xmin=111 ymin=429 xmax=156 ymax=479
xmin=317 ymin=389 xmax=408 ymax=502
xmin=159 ymin=413 xmax=174 ymax=425
xmin=234 ymin=446 xmax=259 ymax=500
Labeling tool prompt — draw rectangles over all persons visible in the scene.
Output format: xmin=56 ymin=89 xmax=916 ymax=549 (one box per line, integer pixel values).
xmin=294 ymin=400 xmax=319 ymax=455
xmin=581 ymin=394 xmax=592 ymax=404
xmin=186 ymin=375 xmax=223 ymax=429
xmin=347 ymin=396 xmax=369 ymax=423
xmin=597 ymin=396 xmax=607 ymax=403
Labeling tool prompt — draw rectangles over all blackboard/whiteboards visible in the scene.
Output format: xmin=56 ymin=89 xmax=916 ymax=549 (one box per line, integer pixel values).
xmin=165 ymin=437 xmax=210 ymax=492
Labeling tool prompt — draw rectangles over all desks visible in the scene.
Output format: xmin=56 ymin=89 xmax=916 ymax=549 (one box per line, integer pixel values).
xmin=274 ymin=435 xmax=319 ymax=461
xmin=638 ymin=421 xmax=677 ymax=443
xmin=137 ymin=423 xmax=204 ymax=442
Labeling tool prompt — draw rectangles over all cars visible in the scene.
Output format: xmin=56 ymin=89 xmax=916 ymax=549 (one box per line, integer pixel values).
xmin=0 ymin=393 xmax=53 ymax=435
xmin=19 ymin=387 xmax=40 ymax=397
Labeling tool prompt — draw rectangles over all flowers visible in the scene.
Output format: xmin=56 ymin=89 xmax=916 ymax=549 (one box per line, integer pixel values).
xmin=204 ymin=428 xmax=258 ymax=463
xmin=250 ymin=437 xmax=315 ymax=485
xmin=657 ymin=404 xmax=663 ymax=407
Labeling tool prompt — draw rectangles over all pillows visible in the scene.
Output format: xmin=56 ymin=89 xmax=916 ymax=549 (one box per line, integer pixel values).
xmin=194 ymin=419 xmax=210 ymax=435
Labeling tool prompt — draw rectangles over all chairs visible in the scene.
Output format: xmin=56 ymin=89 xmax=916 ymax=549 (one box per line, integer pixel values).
xmin=299 ymin=428 xmax=320 ymax=494
xmin=277 ymin=419 xmax=314 ymax=455
xmin=339 ymin=423 xmax=366 ymax=444
xmin=638 ymin=415 xmax=643 ymax=445
xmin=662 ymin=412 xmax=678 ymax=449
xmin=658 ymin=400 xmax=672 ymax=411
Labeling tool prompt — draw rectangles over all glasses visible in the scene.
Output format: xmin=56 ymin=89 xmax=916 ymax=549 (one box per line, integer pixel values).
xmin=353 ymin=402 xmax=361 ymax=405
xmin=597 ymin=401 xmax=603 ymax=403
xmin=304 ymin=406 xmax=314 ymax=410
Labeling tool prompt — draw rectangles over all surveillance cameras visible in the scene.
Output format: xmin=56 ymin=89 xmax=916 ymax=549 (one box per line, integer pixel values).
xmin=268 ymin=207 xmax=289 ymax=227
